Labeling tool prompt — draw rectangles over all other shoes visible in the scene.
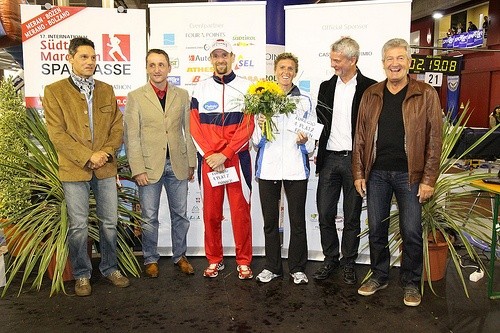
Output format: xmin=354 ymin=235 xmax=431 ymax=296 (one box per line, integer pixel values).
xmin=312 ymin=263 xmax=339 ymax=280
xmin=343 ymin=264 xmax=357 ymax=285
xmin=74 ymin=276 xmax=92 ymax=297
xmin=145 ymin=263 xmax=159 ymax=278
xmin=102 ymin=269 xmax=131 ymax=288
xmin=177 ymin=256 xmax=194 ymax=275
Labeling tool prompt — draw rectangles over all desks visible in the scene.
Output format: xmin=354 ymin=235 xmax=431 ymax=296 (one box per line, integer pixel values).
xmin=454 ymin=176 xmax=499 ymax=300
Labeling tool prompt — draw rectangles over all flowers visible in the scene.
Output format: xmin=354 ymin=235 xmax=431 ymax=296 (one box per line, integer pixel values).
xmin=241 ymin=82 xmax=294 ymax=141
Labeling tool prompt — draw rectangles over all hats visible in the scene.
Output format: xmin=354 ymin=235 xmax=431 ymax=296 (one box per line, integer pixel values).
xmin=469 ymin=22 xmax=472 ymax=23
xmin=209 ymin=38 xmax=233 ymax=55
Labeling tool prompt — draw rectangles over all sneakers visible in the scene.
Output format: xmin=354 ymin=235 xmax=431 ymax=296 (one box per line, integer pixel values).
xmin=289 ymin=271 xmax=308 ymax=284
xmin=403 ymin=283 xmax=422 ymax=306
xmin=202 ymin=261 xmax=225 ymax=277
xmin=255 ymin=269 xmax=281 ymax=283
xmin=357 ymin=278 xmax=388 ymax=296
xmin=236 ymin=264 xmax=254 ymax=279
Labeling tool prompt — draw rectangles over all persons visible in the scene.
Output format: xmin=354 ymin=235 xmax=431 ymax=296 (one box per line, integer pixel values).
xmin=124 ymin=49 xmax=197 ymax=279
xmin=482 ymin=16 xmax=491 ymax=38
xmin=43 ymin=37 xmax=131 ymax=296
xmin=313 ymin=37 xmax=379 ymax=285
xmin=189 ymin=38 xmax=256 ymax=280
xmin=446 ymin=23 xmax=464 ymax=37
xmin=489 ymin=106 xmax=500 ymax=129
xmin=251 ymin=52 xmax=318 ymax=286
xmin=352 ymin=39 xmax=444 ymax=307
xmin=467 ymin=21 xmax=478 ymax=32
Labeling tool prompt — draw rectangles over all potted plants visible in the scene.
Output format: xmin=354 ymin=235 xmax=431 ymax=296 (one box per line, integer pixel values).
xmin=355 ymin=100 xmax=500 ymax=293
xmin=0 ymin=105 xmax=150 ymax=294
xmin=0 ymin=74 xmax=37 ymax=261
